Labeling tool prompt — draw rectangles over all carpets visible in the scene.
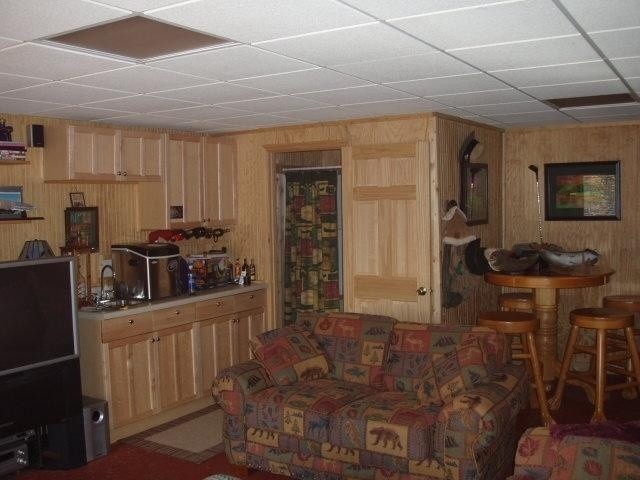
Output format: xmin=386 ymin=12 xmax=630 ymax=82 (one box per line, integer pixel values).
xmin=118 ymin=398 xmax=228 ymax=463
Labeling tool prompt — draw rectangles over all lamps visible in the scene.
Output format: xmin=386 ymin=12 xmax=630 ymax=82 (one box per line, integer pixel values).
xmin=18 ymin=240 xmax=56 ymax=261
xmin=456 ymin=133 xmax=484 ymax=161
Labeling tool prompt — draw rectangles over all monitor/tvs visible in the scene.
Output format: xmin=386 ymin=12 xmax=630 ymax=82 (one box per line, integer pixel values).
xmin=0 ymin=256 xmax=80 ymax=377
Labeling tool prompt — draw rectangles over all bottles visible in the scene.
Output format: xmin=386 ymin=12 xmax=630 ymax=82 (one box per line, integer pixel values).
xmin=77 ymin=264 xmax=87 ymax=308
xmin=235 ymin=258 xmax=256 ymax=284
xmin=187 ymin=265 xmax=196 ymax=296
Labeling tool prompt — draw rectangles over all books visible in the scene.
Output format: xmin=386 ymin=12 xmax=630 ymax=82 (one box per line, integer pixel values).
xmin=1 ymin=141 xmax=29 ymax=161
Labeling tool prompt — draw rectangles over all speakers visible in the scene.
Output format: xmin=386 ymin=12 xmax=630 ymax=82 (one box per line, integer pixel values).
xmin=83 ymin=396 xmax=111 ymax=461
xmin=26 ymin=124 xmax=44 ymax=147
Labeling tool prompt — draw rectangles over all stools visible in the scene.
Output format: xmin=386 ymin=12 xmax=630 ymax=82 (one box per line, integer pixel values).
xmin=469 ymin=305 xmax=559 ymax=428
xmin=494 ymin=292 xmax=535 ymax=376
xmin=553 ymin=302 xmax=637 ymax=425
xmin=603 ymin=293 xmax=640 ymax=403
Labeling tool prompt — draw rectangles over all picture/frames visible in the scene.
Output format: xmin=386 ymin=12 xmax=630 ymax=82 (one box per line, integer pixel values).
xmin=542 ymin=158 xmax=622 ymax=223
xmin=462 ymin=160 xmax=491 ymax=227
xmin=65 ymin=205 xmax=101 ymax=255
xmin=69 ymin=192 xmax=86 ymax=209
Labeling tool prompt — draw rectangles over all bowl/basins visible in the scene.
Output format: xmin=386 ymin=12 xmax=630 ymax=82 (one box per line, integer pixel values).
xmin=541 ymin=248 xmax=588 ymax=275
xmin=495 ymin=252 xmax=539 ymax=274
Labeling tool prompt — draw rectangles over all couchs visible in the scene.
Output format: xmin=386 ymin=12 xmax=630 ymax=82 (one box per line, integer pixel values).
xmin=209 ymin=307 xmax=534 ymax=480
xmin=501 ymin=417 xmax=639 ymax=480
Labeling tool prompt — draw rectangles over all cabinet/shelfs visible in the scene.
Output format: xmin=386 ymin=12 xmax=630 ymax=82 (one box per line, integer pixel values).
xmin=1 ymin=358 xmax=87 ymax=478
xmin=133 ymin=132 xmax=238 ymax=232
xmin=79 ymin=301 xmax=202 ymax=444
xmin=0 ymin=157 xmax=33 ymax=225
xmin=193 ymin=284 xmax=268 ymax=412
xmin=40 ymin=124 xmax=122 ymax=187
xmin=121 ymin=128 xmax=166 ymax=186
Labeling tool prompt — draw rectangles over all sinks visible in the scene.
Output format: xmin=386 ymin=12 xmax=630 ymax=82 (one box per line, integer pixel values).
xmin=96 ymin=297 xmax=148 ymax=308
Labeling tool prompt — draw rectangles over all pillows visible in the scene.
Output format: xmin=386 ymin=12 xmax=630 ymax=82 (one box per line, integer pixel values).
xmin=414 ymin=331 xmax=496 ymax=411
xmin=247 ymin=320 xmax=336 ymax=388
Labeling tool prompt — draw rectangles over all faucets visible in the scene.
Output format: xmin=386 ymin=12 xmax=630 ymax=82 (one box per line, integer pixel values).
xmin=100 ymin=264 xmax=116 ymax=299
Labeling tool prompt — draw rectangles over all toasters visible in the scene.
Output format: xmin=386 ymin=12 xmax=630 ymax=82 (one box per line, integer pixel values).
xmin=111 ymin=241 xmax=181 ymax=301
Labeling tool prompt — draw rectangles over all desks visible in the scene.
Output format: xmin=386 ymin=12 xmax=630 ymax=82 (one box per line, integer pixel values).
xmin=479 ymin=264 xmax=616 ymax=412
